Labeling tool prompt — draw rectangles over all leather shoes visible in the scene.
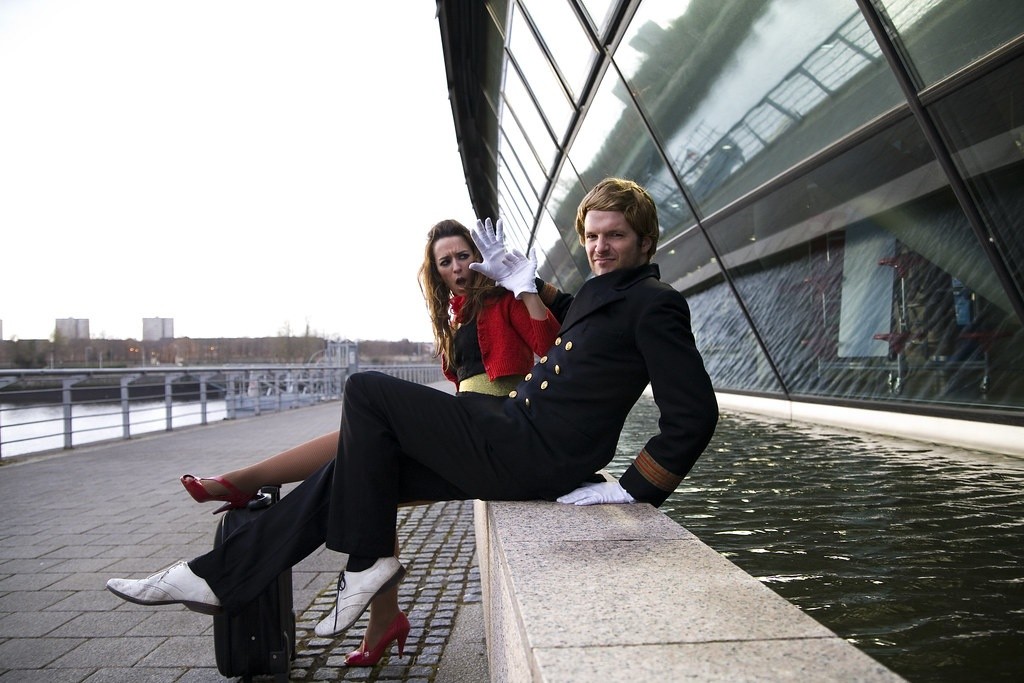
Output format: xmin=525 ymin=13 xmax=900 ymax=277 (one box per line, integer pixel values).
xmin=105 ymin=560 xmax=225 ymax=616
xmin=313 ymin=555 xmax=401 ymax=639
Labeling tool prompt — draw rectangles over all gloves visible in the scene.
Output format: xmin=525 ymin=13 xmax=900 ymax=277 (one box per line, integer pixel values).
xmin=469 ymin=217 xmax=512 ymax=282
xmin=500 ymin=247 xmax=539 ymax=300
xmin=557 ymin=481 xmax=634 ymax=505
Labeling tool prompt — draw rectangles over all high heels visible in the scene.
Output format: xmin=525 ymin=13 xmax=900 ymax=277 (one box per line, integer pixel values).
xmin=343 ymin=611 xmax=411 ymax=666
xmin=180 ymin=473 xmax=257 ymax=515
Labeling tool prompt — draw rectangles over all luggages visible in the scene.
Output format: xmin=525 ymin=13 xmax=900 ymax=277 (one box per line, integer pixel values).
xmin=213 ymin=484 xmax=296 ymax=683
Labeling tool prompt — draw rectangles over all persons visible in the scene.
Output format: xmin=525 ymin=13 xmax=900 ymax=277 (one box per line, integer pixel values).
xmin=179 ymin=220 xmax=559 ymax=666
xmin=107 ymin=178 xmax=720 ymax=638
